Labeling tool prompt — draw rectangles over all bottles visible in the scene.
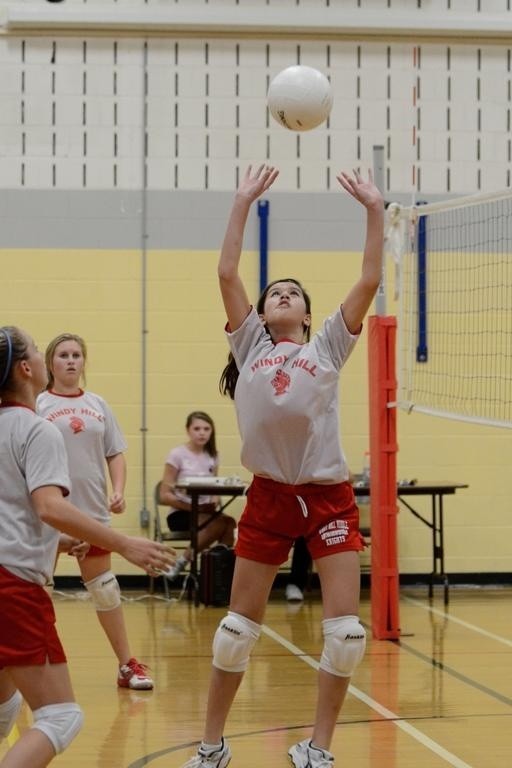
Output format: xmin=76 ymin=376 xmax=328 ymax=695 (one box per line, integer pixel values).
xmin=362 ymin=450 xmax=372 ymax=488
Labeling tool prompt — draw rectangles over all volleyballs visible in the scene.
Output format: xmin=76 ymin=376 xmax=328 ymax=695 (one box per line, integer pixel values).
xmin=266 ymin=66 xmax=333 ymax=131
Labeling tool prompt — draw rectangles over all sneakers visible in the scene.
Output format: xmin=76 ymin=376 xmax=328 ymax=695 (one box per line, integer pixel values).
xmin=180 ymin=738 xmax=231 ymax=768
xmin=285 ymin=584 xmax=304 ymax=600
xmin=157 ymin=559 xmax=185 ymax=578
xmin=117 ymin=657 xmax=154 ymax=690
xmin=288 ymin=739 xmax=334 ymax=768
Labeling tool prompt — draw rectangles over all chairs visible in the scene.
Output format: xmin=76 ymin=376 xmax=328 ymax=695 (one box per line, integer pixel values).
xmin=150 ymin=481 xmax=212 ymax=599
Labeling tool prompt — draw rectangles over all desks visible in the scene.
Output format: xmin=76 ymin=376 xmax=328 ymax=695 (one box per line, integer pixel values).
xmin=174 ymin=480 xmax=468 ymax=608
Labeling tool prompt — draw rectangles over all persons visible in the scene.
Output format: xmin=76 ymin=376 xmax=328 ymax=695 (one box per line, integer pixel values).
xmin=180 ymin=159 xmax=387 ymax=766
xmin=156 ymin=413 xmax=245 ymax=584
xmin=0 ymin=323 xmax=178 ymax=768
xmin=33 ymin=333 xmax=156 ymax=690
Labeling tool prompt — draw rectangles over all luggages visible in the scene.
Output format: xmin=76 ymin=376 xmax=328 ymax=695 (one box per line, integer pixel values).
xmin=198 ymin=545 xmax=236 ymax=608
